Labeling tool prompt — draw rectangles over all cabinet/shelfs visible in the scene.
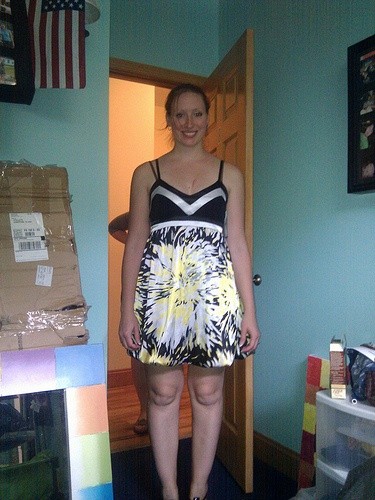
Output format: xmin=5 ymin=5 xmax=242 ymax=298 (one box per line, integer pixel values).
xmin=316 ymin=389 xmax=375 ymax=500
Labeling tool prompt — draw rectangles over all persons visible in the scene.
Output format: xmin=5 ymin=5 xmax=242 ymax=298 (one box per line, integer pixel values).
xmin=118 ymin=83 xmax=260 ymax=500
xmin=0 ymin=23 xmax=15 ymax=48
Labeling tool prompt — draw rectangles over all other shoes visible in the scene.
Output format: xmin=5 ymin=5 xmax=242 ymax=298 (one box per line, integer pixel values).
xmin=134 ymin=418 xmax=149 ymax=435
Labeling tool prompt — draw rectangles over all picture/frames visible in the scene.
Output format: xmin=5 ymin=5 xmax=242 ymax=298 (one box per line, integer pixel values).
xmin=347 ymin=30 xmax=375 ymax=196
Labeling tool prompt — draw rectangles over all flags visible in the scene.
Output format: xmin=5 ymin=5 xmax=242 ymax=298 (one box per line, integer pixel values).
xmin=25 ymin=0 xmax=86 ymax=88
